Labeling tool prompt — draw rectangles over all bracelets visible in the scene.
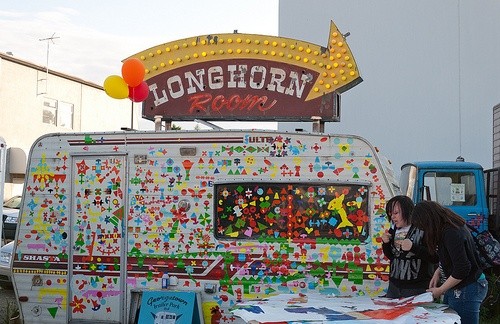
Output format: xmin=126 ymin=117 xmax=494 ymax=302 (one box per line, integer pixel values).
xmin=438 ymin=287 xmax=445 ymax=294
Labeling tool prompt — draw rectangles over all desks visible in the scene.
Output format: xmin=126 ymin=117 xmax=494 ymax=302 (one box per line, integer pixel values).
xmin=127 ymin=287 xmax=206 ymax=324
xmin=232 ymin=296 xmax=461 ymax=324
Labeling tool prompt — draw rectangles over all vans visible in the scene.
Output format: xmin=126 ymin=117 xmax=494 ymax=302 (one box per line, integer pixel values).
xmin=396 ymin=156 xmax=489 ymax=236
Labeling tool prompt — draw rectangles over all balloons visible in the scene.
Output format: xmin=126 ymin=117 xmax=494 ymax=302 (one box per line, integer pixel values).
xmin=128 ymin=81 xmax=150 ymax=102
xmin=104 ymin=75 xmax=129 ymax=99
xmin=121 ymin=58 xmax=145 ymax=87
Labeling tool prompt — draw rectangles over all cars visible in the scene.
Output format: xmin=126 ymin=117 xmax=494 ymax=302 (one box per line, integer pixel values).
xmin=0 ymin=239 xmax=15 ymax=290
xmin=1 ymin=195 xmax=21 ymax=240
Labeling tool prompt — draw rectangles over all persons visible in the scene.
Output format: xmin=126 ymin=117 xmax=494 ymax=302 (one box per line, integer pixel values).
xmin=410 ymin=200 xmax=489 ymax=324
xmin=381 ymin=195 xmax=437 ymax=299
